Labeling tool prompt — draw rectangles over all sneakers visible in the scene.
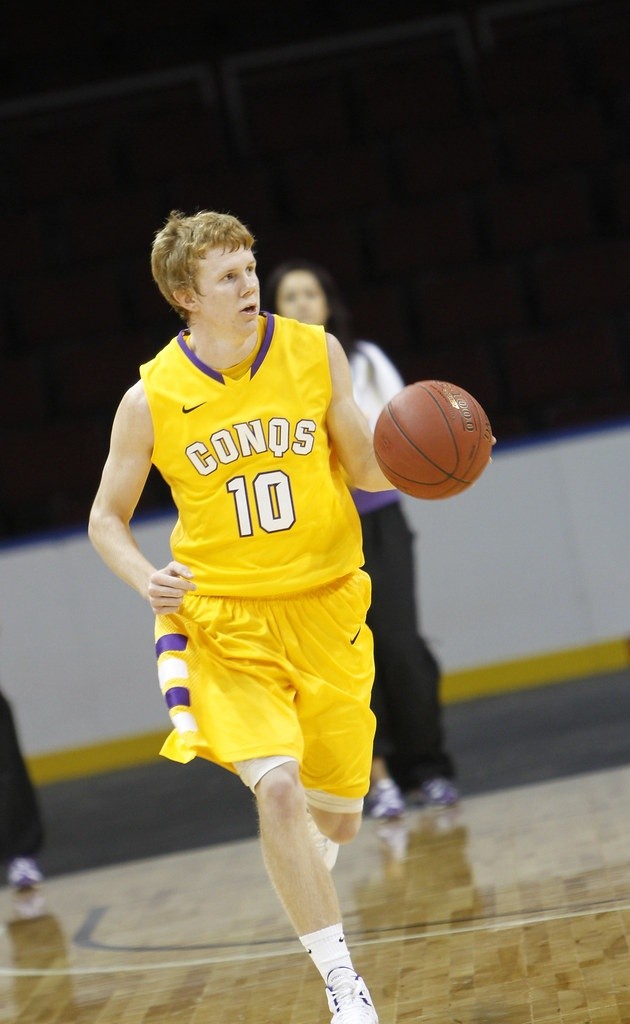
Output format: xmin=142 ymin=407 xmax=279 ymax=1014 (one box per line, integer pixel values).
xmin=325 ymin=974 xmax=379 ymax=1024
xmin=6 ymin=856 xmax=44 ymax=887
xmin=405 ymin=775 xmax=461 ymax=810
xmin=364 ymin=779 xmax=404 ymax=819
xmin=307 ymin=813 xmax=339 ymax=871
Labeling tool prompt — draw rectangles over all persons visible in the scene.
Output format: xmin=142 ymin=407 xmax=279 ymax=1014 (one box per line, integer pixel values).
xmin=0 ymin=692 xmax=41 ymax=889
xmin=87 ymin=210 xmax=496 ymax=1024
xmin=269 ymin=264 xmax=462 ymax=817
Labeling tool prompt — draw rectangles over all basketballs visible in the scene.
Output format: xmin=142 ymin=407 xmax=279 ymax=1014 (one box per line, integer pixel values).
xmin=372 ymin=376 xmax=492 ymax=503
